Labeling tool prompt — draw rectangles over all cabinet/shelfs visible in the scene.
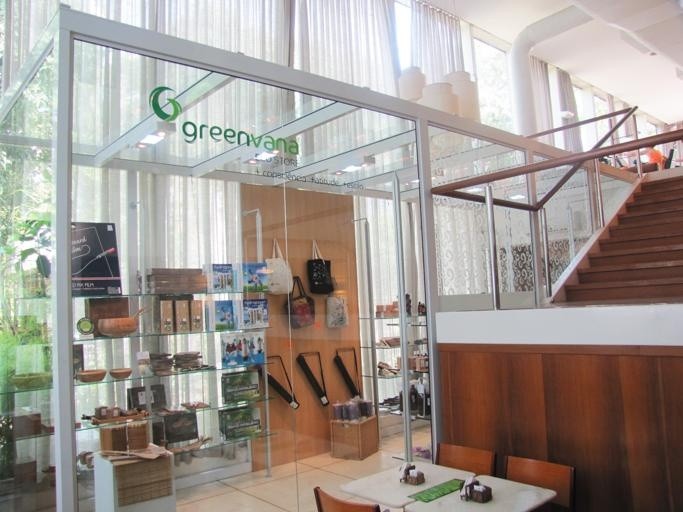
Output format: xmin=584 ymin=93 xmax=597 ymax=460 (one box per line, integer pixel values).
xmin=355 ymin=309 xmax=432 ymax=440
xmin=0 ymin=287 xmax=277 ymax=512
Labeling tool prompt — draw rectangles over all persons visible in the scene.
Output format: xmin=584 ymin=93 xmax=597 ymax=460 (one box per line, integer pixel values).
xmin=639 ymin=145 xmax=664 ymax=170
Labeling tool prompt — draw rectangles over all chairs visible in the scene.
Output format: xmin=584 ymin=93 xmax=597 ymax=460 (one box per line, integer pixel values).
xmin=312 ymin=484 xmax=384 ymax=512
xmin=433 ymin=440 xmax=499 ymax=479
xmin=504 ymin=453 xmax=577 ymax=511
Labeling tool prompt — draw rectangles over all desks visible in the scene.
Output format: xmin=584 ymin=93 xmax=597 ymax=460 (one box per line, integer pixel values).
xmin=335 ymin=455 xmax=558 ymax=512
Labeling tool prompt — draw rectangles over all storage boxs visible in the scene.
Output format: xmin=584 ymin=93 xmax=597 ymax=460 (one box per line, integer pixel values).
xmin=324 ymin=414 xmax=381 ymax=461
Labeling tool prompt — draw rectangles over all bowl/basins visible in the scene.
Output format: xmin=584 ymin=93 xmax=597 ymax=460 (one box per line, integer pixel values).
xmin=78 ymin=370 xmax=106 ymax=382
xmin=109 ymin=368 xmax=131 ymax=379
xmin=96 ymin=316 xmax=139 ymax=338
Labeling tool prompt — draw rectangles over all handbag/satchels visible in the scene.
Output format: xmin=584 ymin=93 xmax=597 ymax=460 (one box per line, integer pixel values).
xmin=286 ymin=276 xmax=315 ymax=329
xmin=265 ymin=238 xmax=293 ymax=295
xmin=326 ymin=276 xmax=348 ymax=328
xmin=307 ymin=240 xmax=334 ymax=293
